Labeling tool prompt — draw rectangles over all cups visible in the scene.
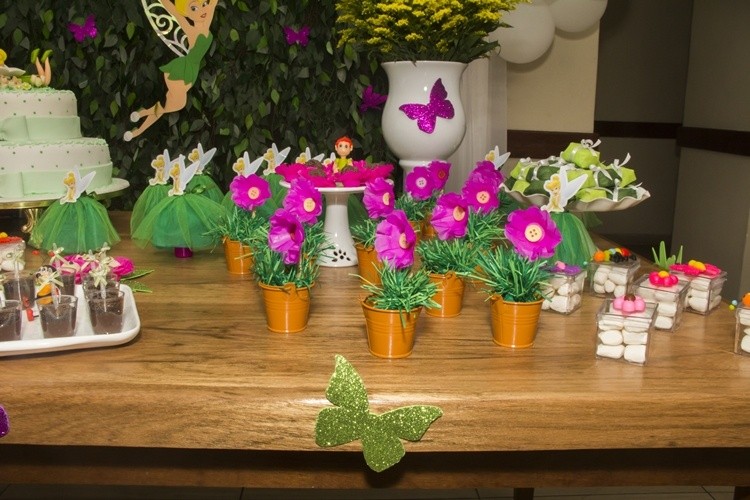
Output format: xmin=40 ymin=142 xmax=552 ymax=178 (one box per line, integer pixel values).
xmin=668 ymin=268 xmax=729 ymax=316
xmin=81 ymin=273 xmax=121 ymax=290
xmin=587 ymin=255 xmax=642 ymax=300
xmin=1 ymin=270 xmax=36 ymax=309
xmin=732 ymin=301 xmax=750 ymax=357
xmin=630 ymin=273 xmax=691 ymax=334
xmin=0 ymin=299 xmax=22 ymax=342
xmin=85 ymin=290 xmax=125 ymax=335
xmin=540 ymin=265 xmax=587 ymax=315
xmin=35 ymin=294 xmax=78 ymax=338
xmin=594 ymin=297 xmax=659 ymax=367
xmin=52 ymin=266 xmax=77 ymax=296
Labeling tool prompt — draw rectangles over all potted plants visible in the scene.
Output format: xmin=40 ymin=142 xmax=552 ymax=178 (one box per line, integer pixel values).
xmin=347 ymin=256 xmax=442 ymax=359
xmin=350 ymin=218 xmax=384 ymax=285
xmin=415 ymin=238 xmax=480 ymax=317
xmin=394 ymin=192 xmax=426 ymax=241
xmin=466 ymin=206 xmax=506 ymax=284
xmin=201 ymin=206 xmax=270 ymax=273
xmin=251 ymin=244 xmax=321 ymax=333
xmin=424 ymin=196 xmax=437 ymax=239
xmin=479 ymin=244 xmax=554 ymax=349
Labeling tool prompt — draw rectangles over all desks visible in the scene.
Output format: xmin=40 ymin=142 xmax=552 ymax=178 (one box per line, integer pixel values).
xmin=0 ymin=210 xmax=750 ymax=500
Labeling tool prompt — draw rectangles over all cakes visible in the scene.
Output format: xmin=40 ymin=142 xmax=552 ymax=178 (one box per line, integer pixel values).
xmin=0 ymin=49 xmax=113 ymax=202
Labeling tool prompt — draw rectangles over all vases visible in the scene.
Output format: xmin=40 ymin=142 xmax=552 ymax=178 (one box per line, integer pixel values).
xmin=381 ymin=61 xmax=469 ymax=161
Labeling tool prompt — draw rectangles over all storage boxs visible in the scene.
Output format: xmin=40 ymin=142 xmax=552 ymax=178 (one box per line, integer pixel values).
xmin=589 ymin=259 xmax=641 ymax=299
xmin=670 ymin=270 xmax=727 ymax=316
xmin=541 ymin=265 xmax=587 ymax=315
xmin=594 ymin=298 xmax=658 ymax=366
xmin=734 ymin=303 xmax=750 ymax=357
xmin=632 ymin=274 xmax=691 ymax=332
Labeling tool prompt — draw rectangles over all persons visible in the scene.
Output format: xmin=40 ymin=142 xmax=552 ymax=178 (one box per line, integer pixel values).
xmin=332 ymin=136 xmax=353 ymax=172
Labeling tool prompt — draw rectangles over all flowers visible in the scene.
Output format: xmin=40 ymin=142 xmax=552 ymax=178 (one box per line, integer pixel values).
xmin=231 ymin=160 xmax=562 ymax=270
xmin=51 ymin=255 xmax=155 ymax=292
xmin=336 ymin=0 xmax=532 ymax=61
